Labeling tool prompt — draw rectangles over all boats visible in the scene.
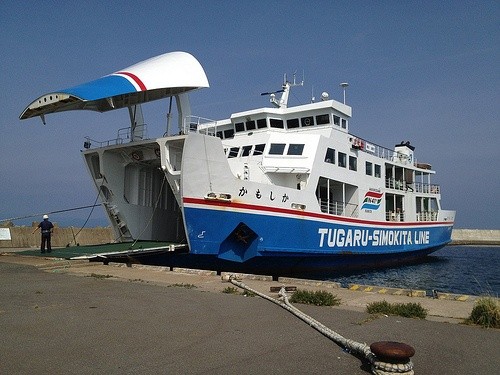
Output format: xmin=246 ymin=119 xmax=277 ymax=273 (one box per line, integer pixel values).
xmin=18 ymin=51 xmax=457 ymax=279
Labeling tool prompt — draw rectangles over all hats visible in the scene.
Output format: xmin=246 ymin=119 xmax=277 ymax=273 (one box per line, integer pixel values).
xmin=43 ymin=215 xmax=49 ymax=220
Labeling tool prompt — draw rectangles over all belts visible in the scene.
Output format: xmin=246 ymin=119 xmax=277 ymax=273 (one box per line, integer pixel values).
xmin=43 ymin=230 xmax=50 ymax=232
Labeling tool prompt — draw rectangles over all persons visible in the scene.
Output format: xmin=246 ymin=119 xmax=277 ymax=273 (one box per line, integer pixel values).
xmin=32 ymin=215 xmax=54 ymax=254
